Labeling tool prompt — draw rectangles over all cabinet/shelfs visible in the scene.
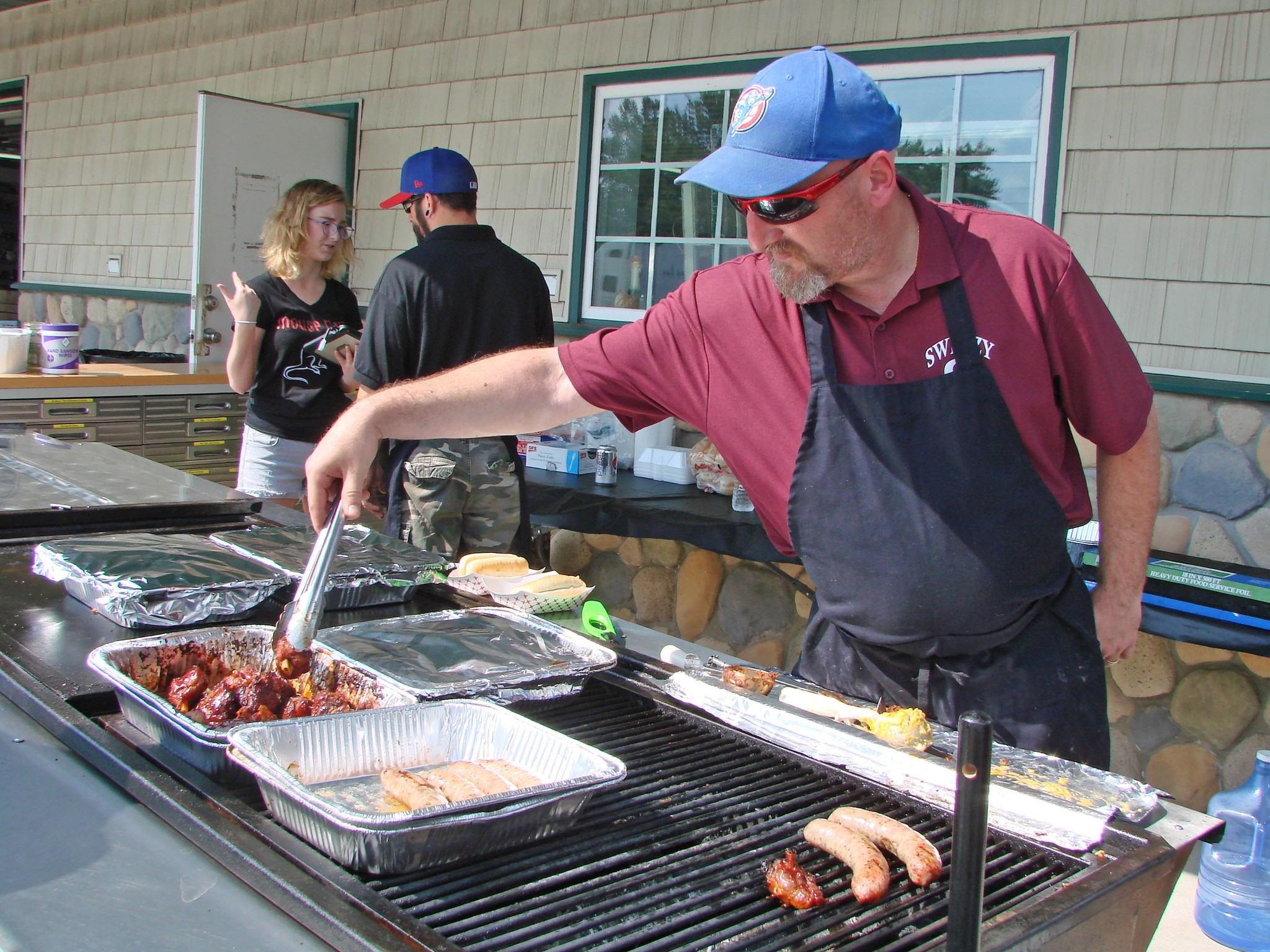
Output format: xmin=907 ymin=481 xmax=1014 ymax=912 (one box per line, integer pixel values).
xmin=1 ymin=394 xmax=249 ymax=488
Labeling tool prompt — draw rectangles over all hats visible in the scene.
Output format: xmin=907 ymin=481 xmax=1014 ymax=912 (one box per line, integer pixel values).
xmin=379 ymin=147 xmax=478 ymax=208
xmin=675 ymin=46 xmax=902 ymax=199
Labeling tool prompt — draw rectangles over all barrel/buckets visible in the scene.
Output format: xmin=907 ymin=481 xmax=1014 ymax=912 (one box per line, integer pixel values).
xmin=0 ymin=321 xmax=50 ymax=373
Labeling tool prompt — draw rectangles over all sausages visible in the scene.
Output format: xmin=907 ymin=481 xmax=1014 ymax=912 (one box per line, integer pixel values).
xmin=827 ymin=806 xmax=942 ymax=887
xmin=802 ymin=818 xmax=890 ymax=904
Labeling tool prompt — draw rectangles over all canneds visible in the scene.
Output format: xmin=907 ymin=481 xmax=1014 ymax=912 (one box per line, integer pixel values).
xmin=594 ymin=444 xmax=618 ymax=486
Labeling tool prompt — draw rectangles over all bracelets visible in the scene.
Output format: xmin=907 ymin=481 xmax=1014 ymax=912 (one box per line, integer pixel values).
xmin=232 ymin=319 xmax=259 ymax=326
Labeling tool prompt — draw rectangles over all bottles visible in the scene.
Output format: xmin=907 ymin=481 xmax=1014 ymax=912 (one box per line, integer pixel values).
xmin=732 ymin=478 xmax=755 ymax=511
xmin=1194 ymin=749 xmax=1270 ymax=952
xmin=42 ymin=323 xmax=79 ymax=375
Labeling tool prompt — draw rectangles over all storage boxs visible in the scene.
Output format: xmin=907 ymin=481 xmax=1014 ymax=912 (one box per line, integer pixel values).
xmin=516 ymin=434 xmax=597 ymax=474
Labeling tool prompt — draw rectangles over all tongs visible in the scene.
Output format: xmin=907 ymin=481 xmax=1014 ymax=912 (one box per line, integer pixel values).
xmin=781 ymin=685 xmax=934 ymax=752
xmin=270 ymin=493 xmax=343 ymax=651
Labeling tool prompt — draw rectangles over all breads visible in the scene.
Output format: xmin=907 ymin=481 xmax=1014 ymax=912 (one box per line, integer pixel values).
xmin=511 ymin=574 xmax=587 ymax=597
xmin=689 ymin=436 xmax=737 ymax=494
xmin=458 ymin=551 xmax=529 ymax=577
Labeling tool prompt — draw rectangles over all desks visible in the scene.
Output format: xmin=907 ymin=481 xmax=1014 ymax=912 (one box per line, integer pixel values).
xmin=520 ymin=457 xmax=817 ymax=599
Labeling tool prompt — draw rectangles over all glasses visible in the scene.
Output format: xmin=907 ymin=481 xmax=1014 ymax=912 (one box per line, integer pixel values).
xmin=726 ymin=156 xmax=868 ymax=225
xmin=307 ymin=217 xmax=354 ymax=241
xmin=402 ymin=194 xmax=424 ymax=214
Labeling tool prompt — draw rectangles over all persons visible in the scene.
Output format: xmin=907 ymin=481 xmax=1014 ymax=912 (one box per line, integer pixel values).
xmin=304 ymin=47 xmax=1161 ymax=772
xmin=353 ymin=147 xmax=556 ymax=563
xmin=215 ymin=180 xmax=363 ymax=510
